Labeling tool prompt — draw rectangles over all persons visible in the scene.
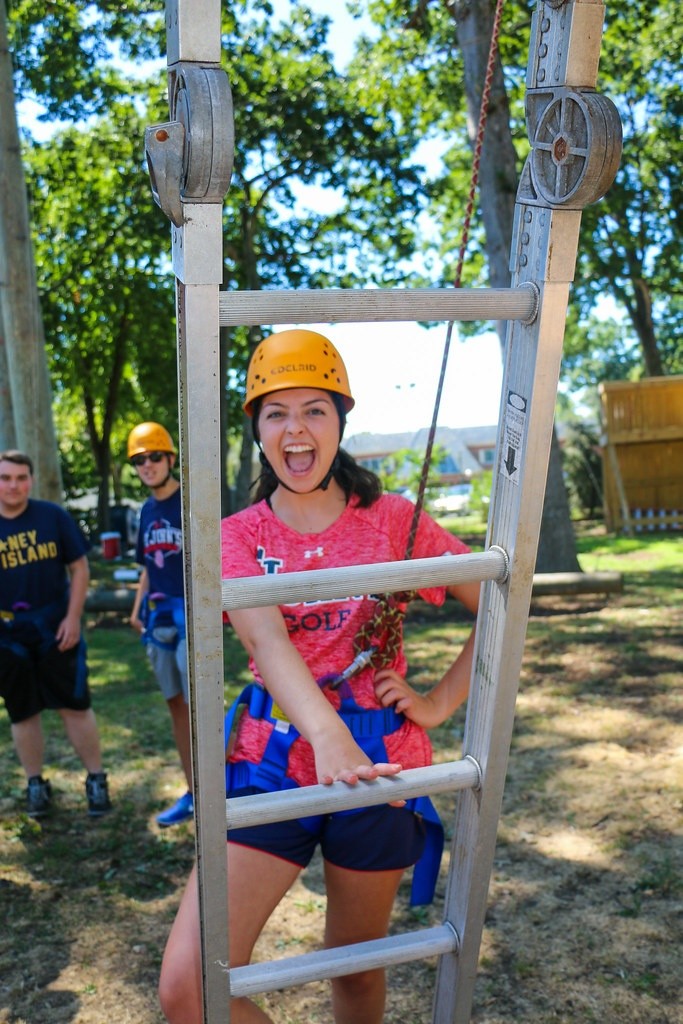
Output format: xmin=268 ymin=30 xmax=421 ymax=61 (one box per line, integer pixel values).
xmin=126 ymin=421 xmax=196 ymax=827
xmin=0 ymin=449 xmax=115 ymax=818
xmin=158 ymin=327 xmax=486 ymax=1024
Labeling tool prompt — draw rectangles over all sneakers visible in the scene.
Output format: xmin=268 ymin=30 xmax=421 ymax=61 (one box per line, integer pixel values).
xmin=25 ymin=776 xmax=53 ymax=816
xmin=85 ymin=773 xmax=112 ymax=816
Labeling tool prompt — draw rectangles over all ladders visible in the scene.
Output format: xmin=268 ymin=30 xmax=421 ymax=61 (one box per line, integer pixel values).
xmin=142 ymin=0 xmax=623 ymax=1024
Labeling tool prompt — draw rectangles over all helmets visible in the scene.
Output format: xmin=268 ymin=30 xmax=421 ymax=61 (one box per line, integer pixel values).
xmin=127 ymin=421 xmax=178 ymax=456
xmin=241 ymin=330 xmax=355 ymax=414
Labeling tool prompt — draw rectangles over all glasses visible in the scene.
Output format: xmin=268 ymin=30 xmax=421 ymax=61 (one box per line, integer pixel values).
xmin=131 ymin=452 xmax=166 ymax=465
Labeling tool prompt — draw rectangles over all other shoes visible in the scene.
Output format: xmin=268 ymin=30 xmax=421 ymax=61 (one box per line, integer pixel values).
xmin=156 ymin=791 xmax=197 ymax=826
